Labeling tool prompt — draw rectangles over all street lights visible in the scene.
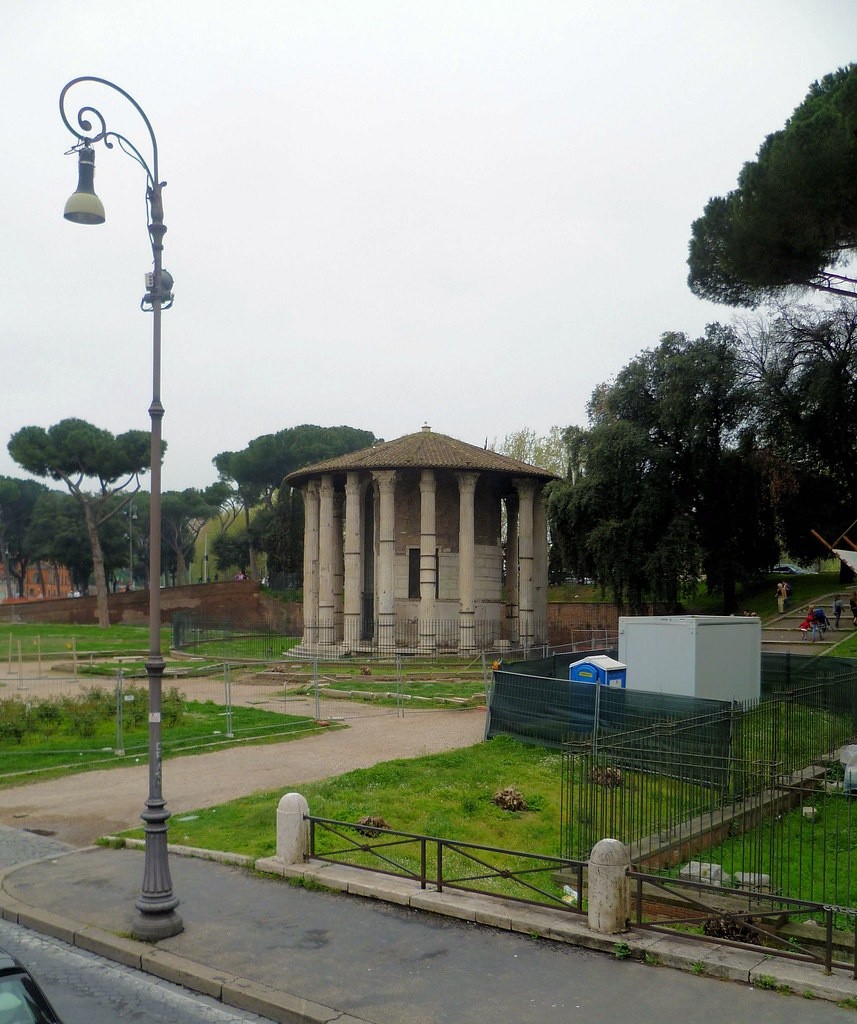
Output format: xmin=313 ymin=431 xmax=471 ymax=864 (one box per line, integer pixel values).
xmin=61 ymin=76 xmax=183 ymax=945
xmin=124 ymin=499 xmax=138 ymax=592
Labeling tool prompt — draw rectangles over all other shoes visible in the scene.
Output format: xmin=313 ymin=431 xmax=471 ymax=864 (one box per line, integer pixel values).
xmin=835 ymin=623 xmax=838 ymax=628
xmin=813 ymin=640 xmax=815 ymax=642
xmin=821 ymin=638 xmax=825 ymax=641
xmin=853 ymin=622 xmax=857 ymax=626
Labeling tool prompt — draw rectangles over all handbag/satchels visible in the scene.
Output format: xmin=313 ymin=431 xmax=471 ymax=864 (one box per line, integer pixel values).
xmin=835 ymin=606 xmax=841 ymax=612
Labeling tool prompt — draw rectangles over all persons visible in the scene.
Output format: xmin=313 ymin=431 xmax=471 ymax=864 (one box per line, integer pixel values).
xmin=743 ymin=610 xmax=751 ymax=617
xmin=850 ymin=591 xmax=857 ymax=626
xmin=69 ymin=591 xmax=80 ymax=598
xmin=126 ymin=583 xmax=132 ymax=591
xmin=799 ymin=605 xmax=830 ymax=642
xmin=833 ymin=594 xmax=845 ymax=628
xmin=775 ymin=580 xmax=790 ymax=614
xmin=235 ymin=570 xmax=250 ymax=580
